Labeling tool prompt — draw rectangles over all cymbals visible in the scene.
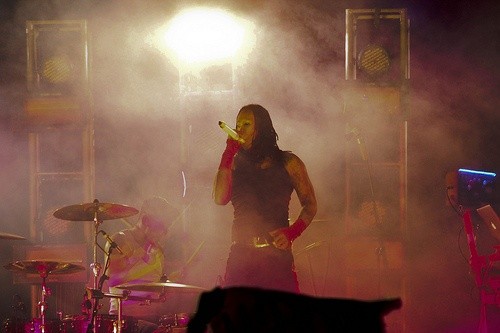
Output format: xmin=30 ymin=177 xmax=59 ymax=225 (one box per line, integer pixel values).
xmin=0 ymin=231 xmax=26 ymax=242
xmin=7 ymin=258 xmax=85 ymax=275
xmin=118 ymin=282 xmax=207 ymax=293
xmin=53 ymin=201 xmax=138 ymax=223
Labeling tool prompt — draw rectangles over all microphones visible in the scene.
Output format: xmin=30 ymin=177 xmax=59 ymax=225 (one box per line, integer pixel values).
xmin=101 ymin=231 xmax=123 ymax=254
xmin=14 ymin=294 xmax=24 ymax=311
xmin=218 ymin=121 xmax=245 ymax=144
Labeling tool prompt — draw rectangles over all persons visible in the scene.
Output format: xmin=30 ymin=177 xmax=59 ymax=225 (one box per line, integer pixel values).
xmin=212 ymin=104 xmax=317 ymax=293
xmin=104 ymin=197 xmax=187 ymax=317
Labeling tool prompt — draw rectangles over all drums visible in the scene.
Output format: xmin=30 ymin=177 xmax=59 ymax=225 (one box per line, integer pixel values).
xmin=62 ymin=311 xmax=135 ymax=333
xmin=14 ymin=315 xmax=62 ymax=333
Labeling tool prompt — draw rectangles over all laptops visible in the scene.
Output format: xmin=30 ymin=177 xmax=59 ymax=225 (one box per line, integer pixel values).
xmin=477 ymin=205 xmax=500 ymax=242
